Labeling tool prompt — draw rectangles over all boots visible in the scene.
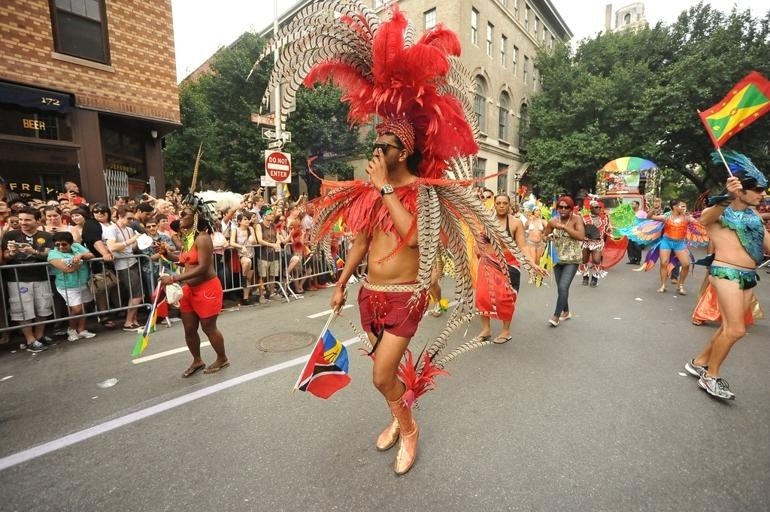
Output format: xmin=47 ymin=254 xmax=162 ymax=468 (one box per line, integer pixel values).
xmin=376 ymin=382 xmax=419 ymax=475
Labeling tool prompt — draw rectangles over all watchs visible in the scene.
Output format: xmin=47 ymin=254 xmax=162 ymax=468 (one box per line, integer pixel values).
xmin=381 ymin=184 xmax=395 ymax=197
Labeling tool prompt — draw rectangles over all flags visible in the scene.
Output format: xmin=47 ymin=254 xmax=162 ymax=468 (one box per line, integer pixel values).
xmin=697 ymin=71 xmax=770 ymax=149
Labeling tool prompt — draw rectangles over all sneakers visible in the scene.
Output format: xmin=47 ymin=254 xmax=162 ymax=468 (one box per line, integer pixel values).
xmin=590 ymin=274 xmax=598 ymax=286
xmin=685 ymin=359 xmax=736 ymax=401
xmin=27 ymin=336 xmax=56 ymax=353
xmin=258 ymin=296 xmax=266 ymax=304
xmin=242 ymin=298 xmax=251 ymax=305
xmin=582 ymin=275 xmax=589 ymax=285
xmin=122 ymin=321 xmax=145 ymax=331
xmin=269 ymin=295 xmax=281 ymax=300
xmin=66 ymin=326 xmax=96 ymax=341
xmin=241 ymin=276 xmax=247 ymax=287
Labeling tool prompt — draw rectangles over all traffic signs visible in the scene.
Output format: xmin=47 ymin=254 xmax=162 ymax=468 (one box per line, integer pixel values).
xmin=268 ymin=138 xmax=284 ymax=150
xmin=262 ymin=128 xmax=291 ymax=143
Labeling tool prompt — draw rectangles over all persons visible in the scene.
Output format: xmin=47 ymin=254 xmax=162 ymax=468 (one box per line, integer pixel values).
xmin=330 ymin=122 xmax=442 ymax=476
xmin=686 ymin=172 xmax=770 ymax=399
xmin=472 ymin=184 xmax=612 ymax=343
xmin=159 ymin=204 xmax=230 ymax=378
xmin=625 ymin=198 xmax=700 ymax=295
xmin=0 ymin=181 xmax=368 ymax=353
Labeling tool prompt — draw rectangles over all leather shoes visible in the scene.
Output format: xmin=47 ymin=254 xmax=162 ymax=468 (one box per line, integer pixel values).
xmin=548 ymin=311 xmax=571 ymax=327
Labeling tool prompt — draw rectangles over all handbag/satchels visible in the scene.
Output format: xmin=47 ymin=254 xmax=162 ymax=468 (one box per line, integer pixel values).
xmin=89 ymin=270 xmax=119 ymax=290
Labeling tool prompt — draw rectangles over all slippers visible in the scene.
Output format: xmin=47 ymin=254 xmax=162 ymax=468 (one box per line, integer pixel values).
xmin=473 ymin=335 xmax=491 ymax=342
xmin=494 ymin=336 xmax=512 ymax=344
xmin=183 ymin=361 xmax=205 ymax=377
xmin=203 ymin=363 xmax=230 ymax=373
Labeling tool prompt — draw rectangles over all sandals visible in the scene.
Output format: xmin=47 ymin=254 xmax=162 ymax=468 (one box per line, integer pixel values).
xmin=677 ymin=283 xmax=687 ymax=294
xmin=657 ymin=285 xmax=667 ymax=292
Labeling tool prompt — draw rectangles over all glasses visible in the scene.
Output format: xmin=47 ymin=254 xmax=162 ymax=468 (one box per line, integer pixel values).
xmin=558 ymin=205 xmax=570 ymax=209
xmin=93 ymin=208 xmax=105 ymax=214
xmin=146 ymin=225 xmax=156 ymax=228
xmin=594 ymin=206 xmax=601 ymax=208
xmin=370 ymin=143 xmax=402 ymax=154
xmin=55 ymin=242 xmax=68 ymax=247
xmin=181 ymin=212 xmax=193 ymax=216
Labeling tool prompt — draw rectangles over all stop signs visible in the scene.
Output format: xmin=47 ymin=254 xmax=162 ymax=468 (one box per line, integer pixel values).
xmin=265 ymin=150 xmax=292 ymax=184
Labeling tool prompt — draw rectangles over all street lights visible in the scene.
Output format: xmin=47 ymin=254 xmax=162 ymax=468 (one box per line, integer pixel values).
xmin=273 ymin=8 xmax=342 ymax=216
